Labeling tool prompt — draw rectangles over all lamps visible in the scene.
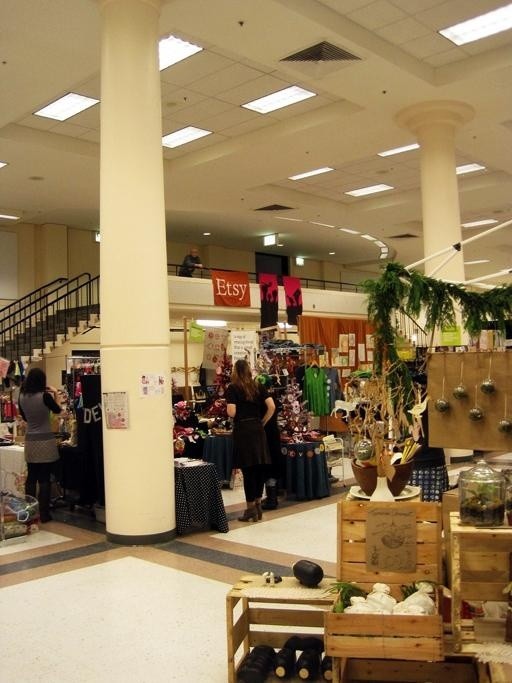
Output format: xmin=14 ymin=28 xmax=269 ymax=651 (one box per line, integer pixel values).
xmin=295 ymin=256 xmax=306 ymax=267
xmin=263 ymin=232 xmax=280 ymax=248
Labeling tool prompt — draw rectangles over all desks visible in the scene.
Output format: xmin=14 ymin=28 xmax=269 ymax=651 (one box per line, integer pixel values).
xmin=0 ymin=439 xmax=34 ymax=502
xmin=51 ymin=435 xmax=233 ymax=538
xmin=281 ymin=432 xmax=332 ymax=501
xmin=189 ymin=431 xmax=233 ymax=491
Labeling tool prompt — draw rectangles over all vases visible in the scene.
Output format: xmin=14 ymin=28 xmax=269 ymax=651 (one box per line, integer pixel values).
xmin=347 ymin=453 xmax=418 ymax=497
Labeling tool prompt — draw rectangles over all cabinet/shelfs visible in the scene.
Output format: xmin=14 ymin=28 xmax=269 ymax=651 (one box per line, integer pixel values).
xmin=222 ymin=572 xmax=350 ymax=682
xmin=324 ymin=436 xmax=346 ymax=491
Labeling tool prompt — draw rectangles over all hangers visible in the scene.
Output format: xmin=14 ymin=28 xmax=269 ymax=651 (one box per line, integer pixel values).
xmin=274 ymin=354 xmax=334 ymax=371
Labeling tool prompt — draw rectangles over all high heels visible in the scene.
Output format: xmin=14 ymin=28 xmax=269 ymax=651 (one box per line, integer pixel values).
xmin=237 ymin=504 xmax=263 ymax=522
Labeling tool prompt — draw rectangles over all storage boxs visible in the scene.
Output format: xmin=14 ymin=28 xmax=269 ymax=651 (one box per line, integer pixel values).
xmin=439 ymin=481 xmax=510 ymax=536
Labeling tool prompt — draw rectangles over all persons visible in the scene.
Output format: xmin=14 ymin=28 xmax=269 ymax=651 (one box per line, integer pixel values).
xmin=55 ymin=400 xmax=77 ymax=500
xmin=177 ymin=246 xmax=203 ymax=278
xmin=253 ymin=370 xmax=278 ymax=510
xmin=226 ymin=358 xmax=277 ymax=522
xmin=17 ymin=365 xmax=62 ymax=523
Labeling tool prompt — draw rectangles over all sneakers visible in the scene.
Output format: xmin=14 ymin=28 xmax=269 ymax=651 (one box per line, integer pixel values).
xmin=40 ymin=513 xmax=53 ymax=523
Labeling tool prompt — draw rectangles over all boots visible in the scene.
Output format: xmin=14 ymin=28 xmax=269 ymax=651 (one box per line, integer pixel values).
xmin=260 ymin=486 xmax=279 ymax=511
